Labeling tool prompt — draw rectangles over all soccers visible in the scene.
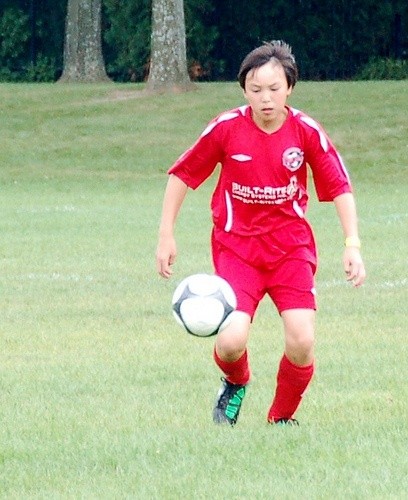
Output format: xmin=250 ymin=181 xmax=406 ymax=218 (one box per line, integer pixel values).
xmin=170 ymin=272 xmax=238 ymax=337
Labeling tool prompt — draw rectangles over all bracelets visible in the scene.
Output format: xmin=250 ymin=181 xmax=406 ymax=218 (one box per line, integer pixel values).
xmin=342 ymin=238 xmax=360 ymax=247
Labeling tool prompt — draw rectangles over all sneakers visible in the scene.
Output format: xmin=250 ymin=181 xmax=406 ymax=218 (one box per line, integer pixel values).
xmin=211 ymin=376 xmax=248 ymax=429
xmin=267 ymin=417 xmax=300 ymax=428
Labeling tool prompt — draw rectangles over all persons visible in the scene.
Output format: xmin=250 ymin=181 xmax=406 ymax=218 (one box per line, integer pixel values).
xmin=155 ymin=40 xmax=366 ymax=425
